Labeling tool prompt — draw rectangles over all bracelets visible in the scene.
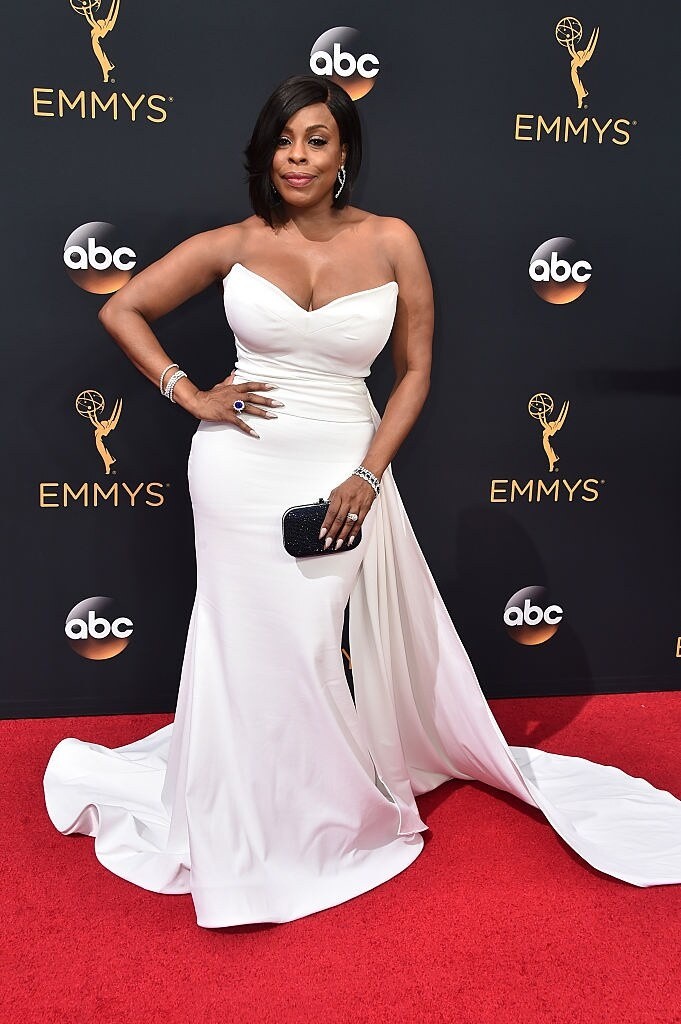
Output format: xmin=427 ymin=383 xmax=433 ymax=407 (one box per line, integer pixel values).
xmin=160 ymin=364 xmax=187 ymax=403
xmin=352 ymin=466 xmax=380 ymax=496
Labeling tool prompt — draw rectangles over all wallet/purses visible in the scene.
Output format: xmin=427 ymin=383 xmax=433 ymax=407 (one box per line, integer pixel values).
xmin=281 ymin=497 xmax=363 ymax=559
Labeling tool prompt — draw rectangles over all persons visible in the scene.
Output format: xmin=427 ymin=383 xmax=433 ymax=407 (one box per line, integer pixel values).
xmin=41 ymin=76 xmax=681 ymax=929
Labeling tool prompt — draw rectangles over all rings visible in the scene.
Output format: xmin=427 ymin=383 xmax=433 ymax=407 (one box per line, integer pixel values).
xmin=234 ymin=400 xmax=245 ymax=413
xmin=347 ymin=513 xmax=358 ymax=521
